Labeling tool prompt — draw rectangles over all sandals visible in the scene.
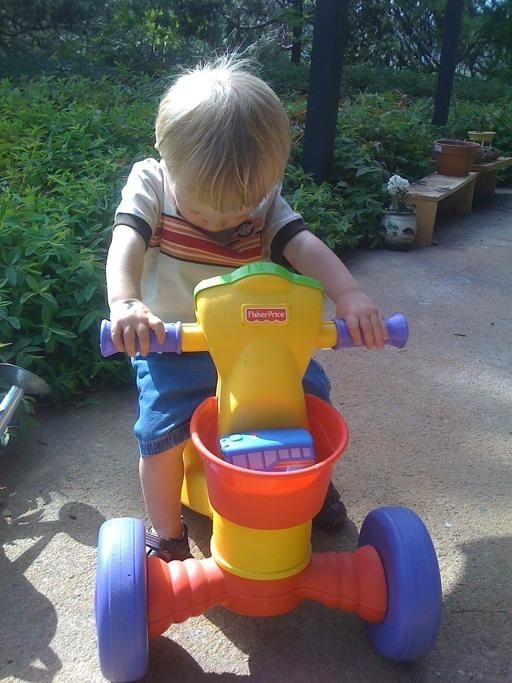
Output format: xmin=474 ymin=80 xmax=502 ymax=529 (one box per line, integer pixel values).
xmin=141 ymin=519 xmax=194 ymax=566
xmin=309 ymin=480 xmax=349 ymax=533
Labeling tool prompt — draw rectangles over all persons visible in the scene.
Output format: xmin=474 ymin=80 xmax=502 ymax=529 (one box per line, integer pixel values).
xmin=102 ymin=40 xmax=389 ymax=563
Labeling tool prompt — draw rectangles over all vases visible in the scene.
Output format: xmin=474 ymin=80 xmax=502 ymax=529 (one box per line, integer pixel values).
xmin=433 ymin=136 xmax=481 ymax=179
xmin=381 ymin=204 xmax=417 ymax=251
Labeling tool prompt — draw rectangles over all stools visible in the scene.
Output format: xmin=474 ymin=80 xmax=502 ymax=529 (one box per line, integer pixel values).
xmin=404 ymin=155 xmax=512 ymax=246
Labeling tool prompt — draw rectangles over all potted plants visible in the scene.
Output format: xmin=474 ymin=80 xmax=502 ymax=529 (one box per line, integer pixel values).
xmin=465 ymin=104 xmax=502 ymax=146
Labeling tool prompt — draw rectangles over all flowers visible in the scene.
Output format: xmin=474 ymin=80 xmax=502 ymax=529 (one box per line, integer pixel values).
xmin=386 ymin=174 xmax=414 ymax=210
